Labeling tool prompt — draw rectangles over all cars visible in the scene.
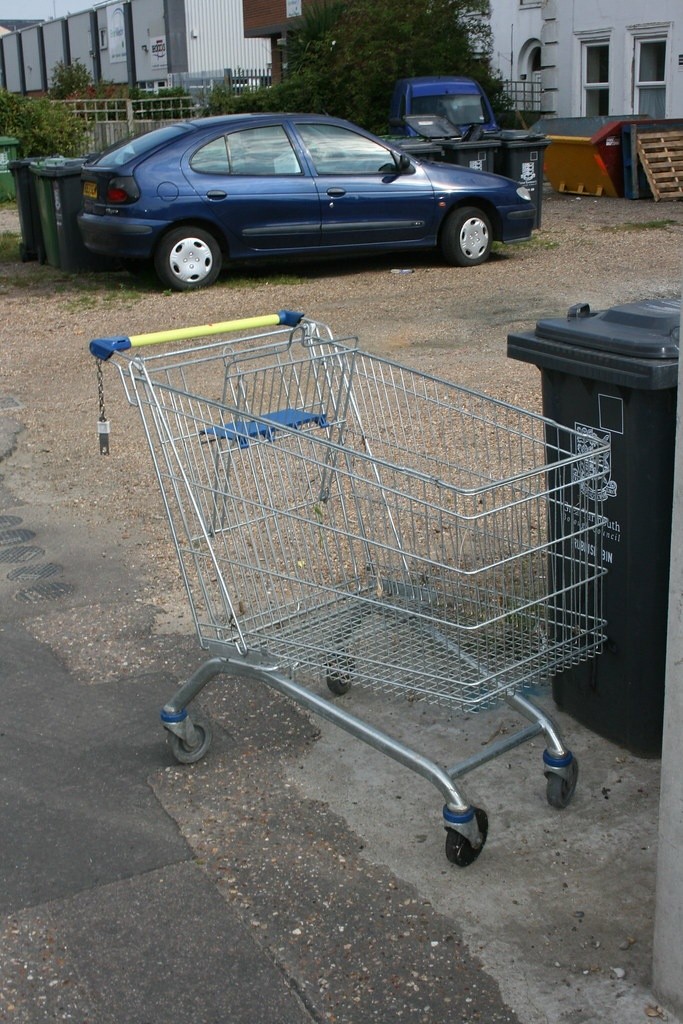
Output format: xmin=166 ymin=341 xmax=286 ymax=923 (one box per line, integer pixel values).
xmin=81 ymin=110 xmax=540 ymax=293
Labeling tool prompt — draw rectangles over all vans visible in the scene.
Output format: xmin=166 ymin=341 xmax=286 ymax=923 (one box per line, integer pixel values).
xmin=391 ymin=77 xmax=498 ymax=138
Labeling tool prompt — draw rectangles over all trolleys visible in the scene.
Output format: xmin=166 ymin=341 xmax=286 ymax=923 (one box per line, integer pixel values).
xmin=87 ymin=310 xmax=615 ymax=873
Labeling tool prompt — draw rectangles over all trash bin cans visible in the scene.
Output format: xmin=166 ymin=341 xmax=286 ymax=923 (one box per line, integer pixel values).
xmin=486 ymin=128 xmax=552 ymax=231
xmin=8 ymin=160 xmax=51 ymax=265
xmin=42 ymin=153 xmax=101 ymax=271
xmin=0 ymin=135 xmax=20 ymax=202
xmin=402 ymin=114 xmax=502 ymax=175
xmin=31 ymin=157 xmax=75 ymax=268
xmin=504 ymin=292 xmax=681 ymax=760
xmin=389 ymin=138 xmax=445 ymax=165
